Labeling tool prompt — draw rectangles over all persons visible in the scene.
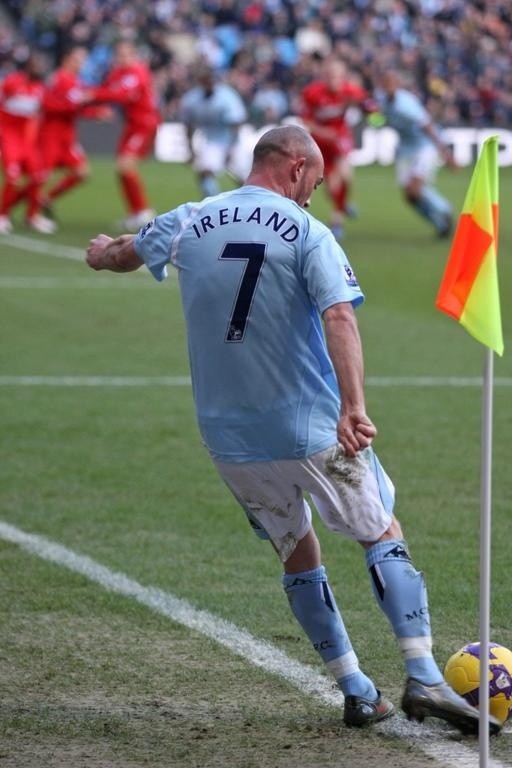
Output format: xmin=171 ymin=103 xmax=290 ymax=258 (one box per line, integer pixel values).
xmin=84 ymin=124 xmax=503 ymax=738
xmin=1 ymin=0 xmax=509 ymax=235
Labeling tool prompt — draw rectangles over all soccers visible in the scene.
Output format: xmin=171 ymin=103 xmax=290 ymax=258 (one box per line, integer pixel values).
xmin=444 ymin=641 xmax=512 ymax=724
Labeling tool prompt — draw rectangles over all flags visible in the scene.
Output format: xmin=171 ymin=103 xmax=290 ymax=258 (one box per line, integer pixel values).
xmin=434 ymin=134 xmax=503 ymax=356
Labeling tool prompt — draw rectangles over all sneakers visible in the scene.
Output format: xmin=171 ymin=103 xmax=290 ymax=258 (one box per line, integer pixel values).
xmin=344 ymin=690 xmax=397 ymax=729
xmin=401 ymin=676 xmax=503 ymax=738
xmin=30 ymin=214 xmax=56 ymax=237
xmin=121 ymin=211 xmax=155 ymax=233
